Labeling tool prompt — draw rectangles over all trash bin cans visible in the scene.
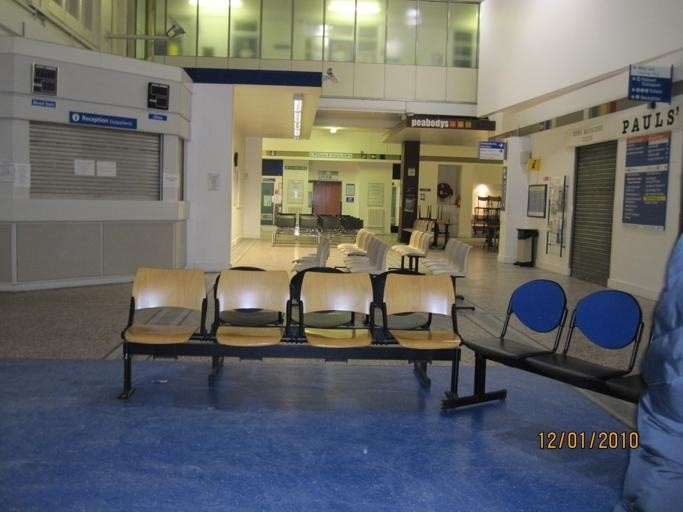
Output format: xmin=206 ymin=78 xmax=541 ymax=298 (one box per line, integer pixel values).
xmin=513 ymin=226 xmax=539 ymax=269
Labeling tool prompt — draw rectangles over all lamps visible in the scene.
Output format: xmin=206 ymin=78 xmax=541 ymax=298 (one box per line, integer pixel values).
xmin=105 ymin=23 xmax=185 ymax=42
xmin=293 ymin=93 xmax=303 ymax=142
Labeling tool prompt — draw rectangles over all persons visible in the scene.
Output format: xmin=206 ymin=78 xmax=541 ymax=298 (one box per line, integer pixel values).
xmin=271 ymin=190 xmax=282 ymax=219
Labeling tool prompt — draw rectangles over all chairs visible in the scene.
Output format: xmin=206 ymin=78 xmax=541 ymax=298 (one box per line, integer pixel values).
xmin=472 ymin=196 xmax=505 ymax=250
xmin=442 ymin=280 xmax=646 ymax=410
xmin=273 ymin=205 xmax=474 ymax=311
xmin=118 ymin=267 xmax=462 ymax=403
